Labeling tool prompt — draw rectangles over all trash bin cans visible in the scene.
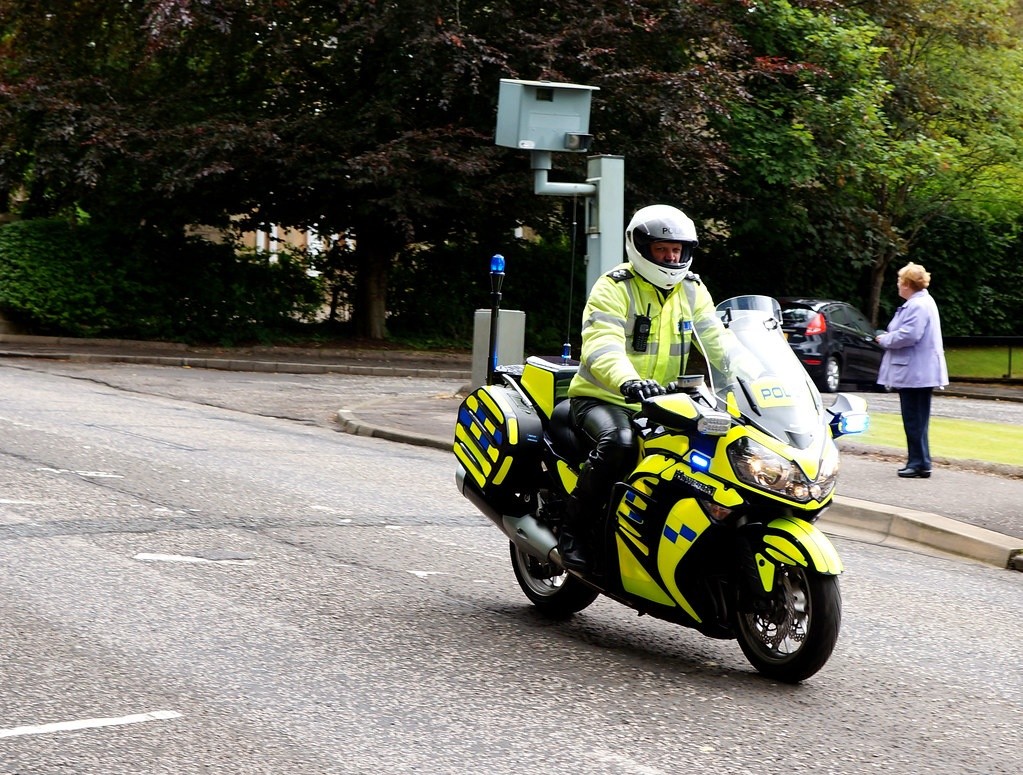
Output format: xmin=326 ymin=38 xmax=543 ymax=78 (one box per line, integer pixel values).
xmin=471 ymin=309 xmax=525 ymax=390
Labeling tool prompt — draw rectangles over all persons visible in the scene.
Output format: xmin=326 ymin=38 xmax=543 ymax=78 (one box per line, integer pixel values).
xmin=873 ymin=261 xmax=950 ymax=477
xmin=557 ymin=203 xmax=771 ymax=568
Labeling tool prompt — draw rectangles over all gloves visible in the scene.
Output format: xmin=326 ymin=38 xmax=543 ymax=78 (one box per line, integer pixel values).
xmin=620 ymin=379 xmax=666 ymax=402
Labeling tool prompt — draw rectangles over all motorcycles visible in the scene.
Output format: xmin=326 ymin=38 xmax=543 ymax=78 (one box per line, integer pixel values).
xmin=449 ymin=254 xmax=868 ymax=685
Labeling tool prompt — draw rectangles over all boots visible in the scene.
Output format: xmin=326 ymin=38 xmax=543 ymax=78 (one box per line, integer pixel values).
xmin=558 ymin=450 xmax=612 ymax=572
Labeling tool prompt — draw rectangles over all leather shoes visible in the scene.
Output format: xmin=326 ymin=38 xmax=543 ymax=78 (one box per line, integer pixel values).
xmin=898 ymin=467 xmax=931 ymax=477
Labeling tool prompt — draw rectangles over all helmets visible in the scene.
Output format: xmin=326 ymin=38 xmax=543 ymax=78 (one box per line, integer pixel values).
xmin=625 ymin=205 xmax=697 ymax=290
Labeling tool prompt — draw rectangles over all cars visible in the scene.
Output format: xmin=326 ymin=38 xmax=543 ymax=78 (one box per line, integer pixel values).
xmin=775 ymin=298 xmax=884 ymax=393
